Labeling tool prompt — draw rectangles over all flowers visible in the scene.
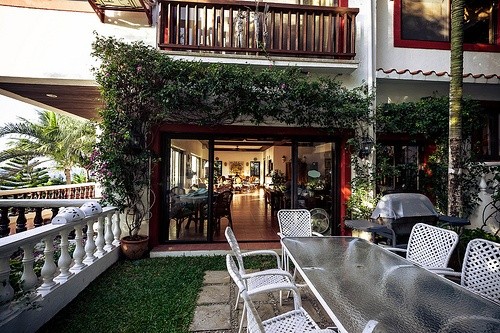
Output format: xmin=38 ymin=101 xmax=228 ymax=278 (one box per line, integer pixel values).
xmin=81 ymin=29 xmax=377 ymax=240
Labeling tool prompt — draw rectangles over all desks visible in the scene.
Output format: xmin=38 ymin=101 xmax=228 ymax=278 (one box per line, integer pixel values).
xmin=173 ymin=193 xmax=219 ymax=239
xmin=264 ymin=187 xmax=286 ymax=227
xmin=280 ymin=234 xmax=500 ymax=333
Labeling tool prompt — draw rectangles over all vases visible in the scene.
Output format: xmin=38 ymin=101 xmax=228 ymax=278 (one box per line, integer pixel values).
xmin=121 ymin=235 xmax=149 ymax=261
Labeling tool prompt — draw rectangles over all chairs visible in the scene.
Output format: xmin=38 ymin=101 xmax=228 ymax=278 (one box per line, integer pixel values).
xmin=209 ymin=191 xmax=233 ymax=235
xmin=170 ymin=186 xmax=500 ymax=333
